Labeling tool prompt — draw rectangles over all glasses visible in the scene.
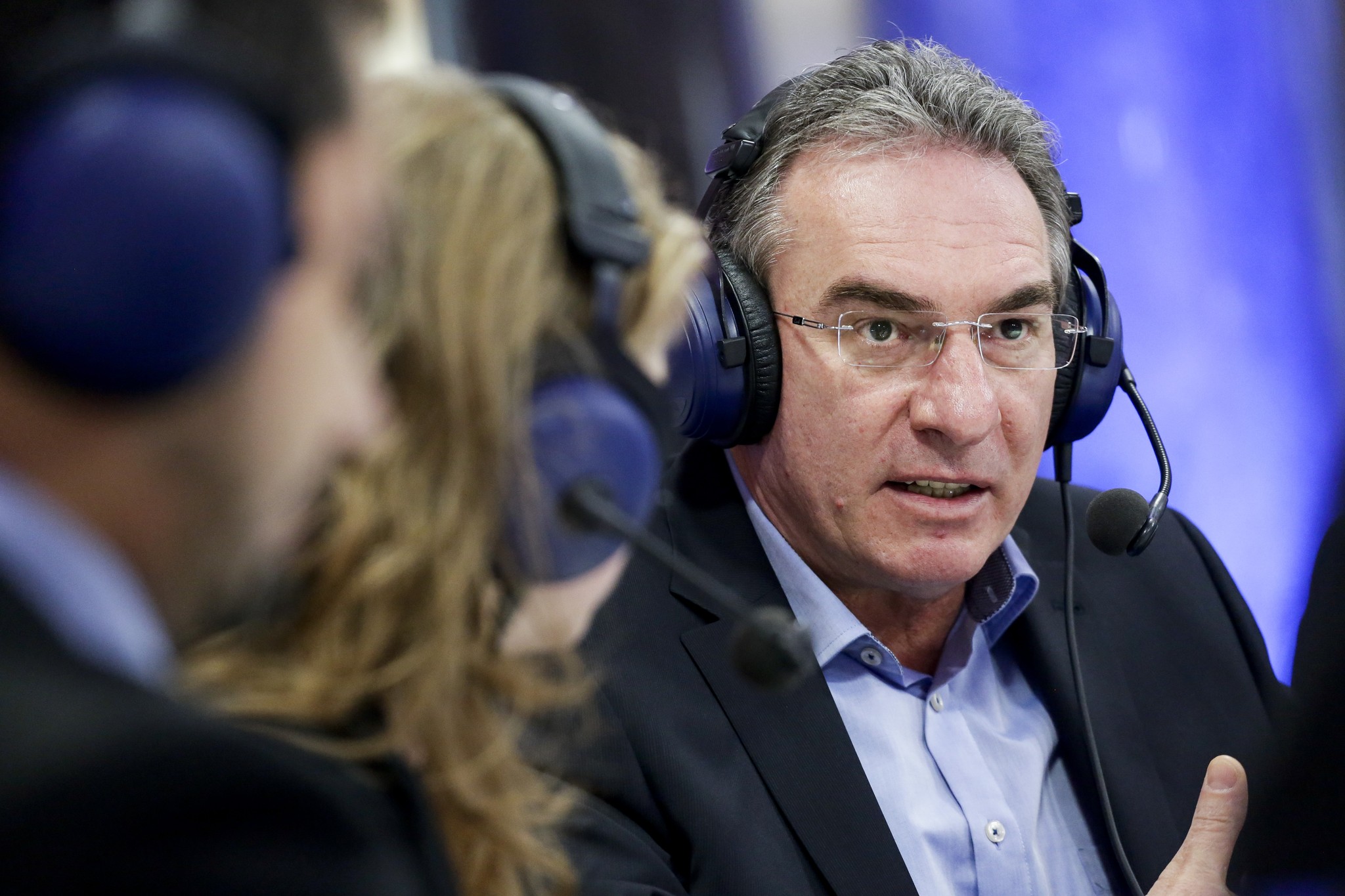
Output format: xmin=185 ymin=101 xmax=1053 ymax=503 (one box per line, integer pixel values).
xmin=770 ymin=310 xmax=1088 ymax=370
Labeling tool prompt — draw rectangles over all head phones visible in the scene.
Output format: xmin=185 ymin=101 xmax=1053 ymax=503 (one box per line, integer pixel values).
xmin=477 ymin=75 xmax=678 ymax=587
xmin=0 ymin=0 xmax=310 ymax=408
xmin=666 ymin=68 xmax=1127 ymax=453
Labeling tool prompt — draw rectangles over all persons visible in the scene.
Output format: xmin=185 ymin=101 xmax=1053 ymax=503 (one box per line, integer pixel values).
xmin=183 ymin=51 xmax=693 ymax=896
xmin=528 ymin=30 xmax=1302 ymax=894
xmin=0 ymin=0 xmax=426 ymax=896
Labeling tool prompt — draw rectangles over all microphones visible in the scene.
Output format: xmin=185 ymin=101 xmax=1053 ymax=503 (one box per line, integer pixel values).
xmin=564 ymin=487 xmax=811 ymax=692
xmin=1085 ymin=369 xmax=1172 ymax=556
